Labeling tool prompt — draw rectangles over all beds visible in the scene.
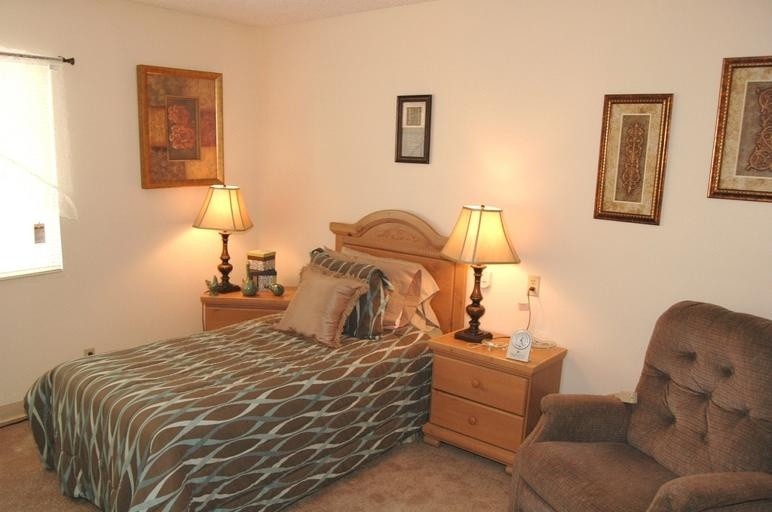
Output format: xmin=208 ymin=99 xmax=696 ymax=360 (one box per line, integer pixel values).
xmin=24 ymin=211 xmax=466 ymax=511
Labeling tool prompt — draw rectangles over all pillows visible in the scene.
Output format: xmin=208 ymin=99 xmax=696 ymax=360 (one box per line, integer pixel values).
xmin=275 ymin=264 xmax=370 ymax=348
xmin=308 ymin=252 xmax=394 ymax=340
xmin=341 ymin=246 xmax=440 ymax=333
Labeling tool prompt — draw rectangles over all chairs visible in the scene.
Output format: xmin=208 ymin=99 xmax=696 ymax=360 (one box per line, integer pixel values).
xmin=505 ymin=300 xmax=771 ymax=512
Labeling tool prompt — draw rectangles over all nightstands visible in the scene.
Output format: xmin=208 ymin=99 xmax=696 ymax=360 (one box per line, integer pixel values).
xmin=199 ymin=285 xmax=300 ymax=332
xmin=423 ymin=328 xmax=568 ymax=477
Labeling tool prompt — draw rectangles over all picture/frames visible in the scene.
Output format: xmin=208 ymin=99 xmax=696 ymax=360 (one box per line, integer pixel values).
xmin=705 ymin=54 xmax=772 ymax=202
xmin=594 ymin=93 xmax=673 ymax=225
xmin=137 ymin=64 xmax=225 ymax=188
xmin=395 ymin=95 xmax=432 ymax=164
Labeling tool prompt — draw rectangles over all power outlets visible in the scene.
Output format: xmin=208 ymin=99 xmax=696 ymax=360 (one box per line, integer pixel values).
xmin=528 ymin=275 xmax=541 ymax=297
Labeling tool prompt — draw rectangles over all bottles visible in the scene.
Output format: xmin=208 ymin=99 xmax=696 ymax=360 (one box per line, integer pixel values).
xmin=273 ymin=285 xmax=284 ymax=296
xmin=241 ymin=280 xmax=255 ymax=296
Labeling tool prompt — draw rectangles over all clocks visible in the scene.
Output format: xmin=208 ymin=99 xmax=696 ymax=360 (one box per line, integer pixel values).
xmin=505 ymin=328 xmax=532 ymax=364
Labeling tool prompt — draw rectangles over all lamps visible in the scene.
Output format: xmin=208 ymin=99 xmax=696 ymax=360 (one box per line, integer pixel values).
xmin=193 ymin=186 xmax=253 ymax=293
xmin=442 ymin=207 xmax=520 ymax=343
